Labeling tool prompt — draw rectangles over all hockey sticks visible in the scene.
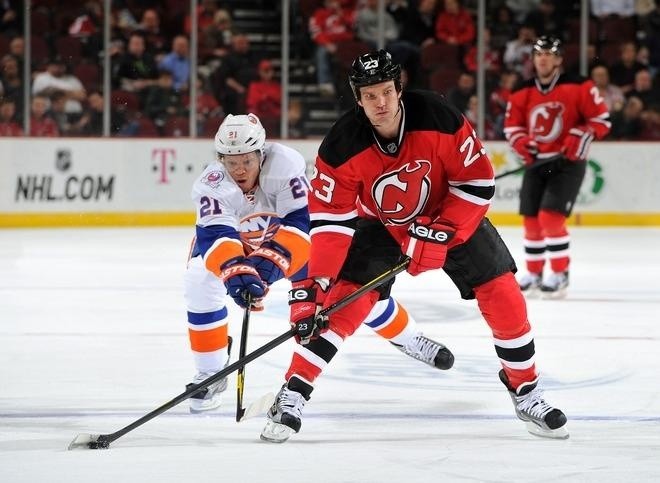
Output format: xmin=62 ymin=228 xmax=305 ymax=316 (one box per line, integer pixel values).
xmin=67 ymin=257 xmax=411 ymax=453
xmin=236 ymin=289 xmax=278 ymax=421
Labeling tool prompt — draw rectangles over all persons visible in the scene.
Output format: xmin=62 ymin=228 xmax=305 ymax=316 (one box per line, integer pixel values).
xmin=502 ymin=35 xmax=612 ymax=291
xmin=184 ymin=112 xmax=454 ymax=400
xmin=260 ymin=47 xmax=567 ymax=433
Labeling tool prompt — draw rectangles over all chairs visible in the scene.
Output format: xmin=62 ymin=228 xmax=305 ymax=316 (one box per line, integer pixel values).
xmin=0 ymin=0 xmax=297 ymax=138
xmin=298 ymin=0 xmax=659 ymax=141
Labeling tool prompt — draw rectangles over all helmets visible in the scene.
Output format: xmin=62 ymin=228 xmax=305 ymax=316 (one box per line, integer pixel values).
xmin=348 ymin=50 xmax=404 ymax=103
xmin=534 ymin=36 xmax=562 ymax=56
xmin=216 ymin=112 xmax=268 ymax=171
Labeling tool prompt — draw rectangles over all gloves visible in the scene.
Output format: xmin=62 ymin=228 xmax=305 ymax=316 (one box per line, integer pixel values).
xmin=221 ymin=240 xmax=291 ymax=311
xmin=509 ymin=129 xmax=538 ymax=166
xmin=289 ymin=276 xmax=335 ymax=345
xmin=399 ymin=217 xmax=456 ymax=276
xmin=563 ymin=125 xmax=595 ymax=160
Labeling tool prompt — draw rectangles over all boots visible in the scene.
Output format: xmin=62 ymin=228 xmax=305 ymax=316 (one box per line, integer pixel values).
xmin=499 ymin=369 xmax=567 ymax=429
xmin=389 ymin=335 xmax=454 ymax=370
xmin=267 ymin=373 xmax=313 ymax=433
xmin=185 ymin=337 xmax=233 ymax=398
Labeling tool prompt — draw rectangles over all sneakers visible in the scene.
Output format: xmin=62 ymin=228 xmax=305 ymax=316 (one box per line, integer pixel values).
xmin=542 ymin=268 xmax=568 ymax=291
xmin=519 ymin=271 xmax=542 ymax=291
